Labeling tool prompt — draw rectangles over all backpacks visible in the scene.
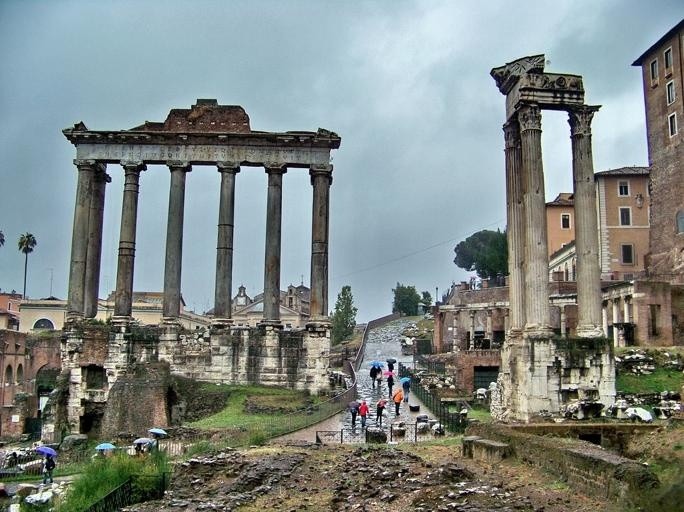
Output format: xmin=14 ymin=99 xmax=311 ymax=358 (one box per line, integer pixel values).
xmin=45 ymin=457 xmax=55 ymax=471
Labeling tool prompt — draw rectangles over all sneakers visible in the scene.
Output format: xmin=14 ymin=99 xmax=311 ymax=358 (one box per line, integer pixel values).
xmin=39 ymin=483 xmax=46 ymax=486
xmin=49 ymin=482 xmax=54 ymax=485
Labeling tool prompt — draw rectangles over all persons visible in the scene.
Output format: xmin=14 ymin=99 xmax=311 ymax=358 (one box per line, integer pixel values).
xmin=91 ymin=449 xmax=106 ymax=462
xmin=144 ymin=433 xmax=160 ymax=452
xmin=135 ymin=443 xmax=143 ymax=457
xmin=351 ymin=358 xmax=410 ymax=428
xmin=40 ymin=453 xmax=54 ymax=485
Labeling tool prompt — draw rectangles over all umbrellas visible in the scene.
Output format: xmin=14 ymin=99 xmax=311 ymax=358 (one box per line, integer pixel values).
xmin=35 ymin=446 xmax=57 ymax=457
xmin=95 ymin=443 xmax=116 ymax=450
xmin=133 ymin=438 xmax=151 ymax=444
xmin=148 ymin=429 xmax=167 ymax=438
